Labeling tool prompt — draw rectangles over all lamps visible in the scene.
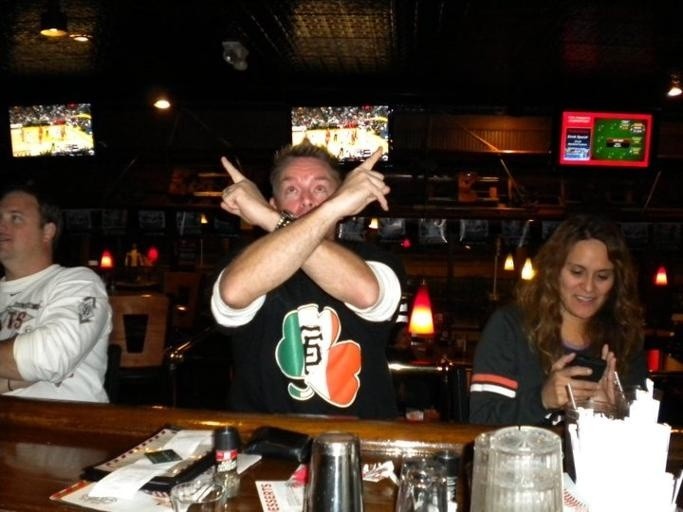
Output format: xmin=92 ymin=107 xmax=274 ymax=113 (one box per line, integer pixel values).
xmin=408 ymin=278 xmax=435 ymax=335
xmin=504 ymin=249 xmax=516 ymax=272
xmin=655 ymin=264 xmax=668 ymax=285
xmin=39 ymin=10 xmax=68 ymax=37
xmin=666 ymin=74 xmax=683 ymax=99
xmin=100 ymin=248 xmax=113 ymax=269
xmin=521 ymin=255 xmax=536 ymax=280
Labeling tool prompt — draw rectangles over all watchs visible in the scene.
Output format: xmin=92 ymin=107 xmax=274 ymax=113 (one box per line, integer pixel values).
xmin=273 ymin=210 xmax=298 ymax=232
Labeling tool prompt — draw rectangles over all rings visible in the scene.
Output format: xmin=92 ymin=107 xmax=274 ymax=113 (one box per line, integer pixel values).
xmin=603 ymin=376 xmax=608 ymax=382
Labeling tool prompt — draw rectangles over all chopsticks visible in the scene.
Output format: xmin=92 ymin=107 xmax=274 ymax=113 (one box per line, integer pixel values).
xmin=566 ymin=378 xmax=677 ymax=512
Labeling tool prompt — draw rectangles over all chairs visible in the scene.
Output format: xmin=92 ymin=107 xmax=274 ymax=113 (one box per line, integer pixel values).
xmin=109 ymin=270 xmax=202 ymax=406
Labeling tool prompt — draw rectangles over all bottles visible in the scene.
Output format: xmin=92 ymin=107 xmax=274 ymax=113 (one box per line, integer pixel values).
xmin=212 ymin=424 xmax=241 ymax=497
xmin=435 ymin=452 xmax=458 ymax=511
xmin=471 ymin=428 xmax=562 ymax=511
xmin=306 ymin=432 xmax=363 ymax=512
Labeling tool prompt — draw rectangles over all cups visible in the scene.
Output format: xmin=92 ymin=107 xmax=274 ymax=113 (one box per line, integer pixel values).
xmin=169 ymin=483 xmax=225 ymax=511
xmin=397 ymin=459 xmax=448 ymax=511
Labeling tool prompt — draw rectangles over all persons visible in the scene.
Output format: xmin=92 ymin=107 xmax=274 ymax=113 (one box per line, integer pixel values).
xmin=0 ymin=183 xmax=112 ymax=407
xmin=10 ymin=104 xmax=94 ymax=157
xmin=207 ymin=138 xmax=413 ymax=419
xmin=439 ymin=168 xmax=486 ymax=202
xmin=163 ymin=168 xmax=201 ymax=196
xmin=468 ymin=210 xmax=636 ymax=426
xmin=292 ymin=105 xmax=389 ymax=161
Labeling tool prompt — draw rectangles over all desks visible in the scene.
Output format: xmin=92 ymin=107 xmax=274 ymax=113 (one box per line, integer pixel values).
xmin=386 ymin=332 xmax=480 ymax=424
